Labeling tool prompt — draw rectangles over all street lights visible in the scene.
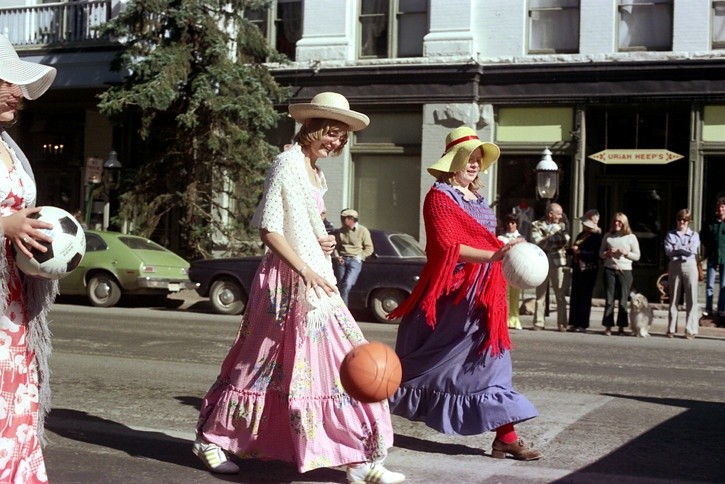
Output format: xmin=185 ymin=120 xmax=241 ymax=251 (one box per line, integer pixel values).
xmin=536 ymin=146 xmax=558 ymax=317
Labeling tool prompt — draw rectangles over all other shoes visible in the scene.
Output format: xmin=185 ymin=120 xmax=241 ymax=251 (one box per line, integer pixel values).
xmin=618 ymin=330 xmax=625 ymax=336
xmin=559 ymin=324 xmax=566 ymax=331
xmin=667 ymin=332 xmax=674 ymax=338
xmin=570 ymin=326 xmax=586 ymax=333
xmin=603 ymin=330 xmax=611 ymax=335
xmin=533 ymin=325 xmax=544 ymax=330
xmin=685 ymin=333 xmax=695 ymax=339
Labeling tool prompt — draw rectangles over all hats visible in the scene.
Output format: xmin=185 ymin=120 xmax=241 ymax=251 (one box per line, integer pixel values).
xmin=340 ymin=208 xmax=359 ymax=222
xmin=426 ymin=126 xmax=501 ymax=178
xmin=289 ymin=92 xmax=370 ymax=132
xmin=0 ymin=34 xmax=56 ymax=100
xmin=579 ymin=209 xmax=600 ymax=229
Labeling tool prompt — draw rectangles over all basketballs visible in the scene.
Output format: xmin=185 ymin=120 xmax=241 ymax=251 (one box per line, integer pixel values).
xmin=340 ymin=341 xmax=403 ymax=404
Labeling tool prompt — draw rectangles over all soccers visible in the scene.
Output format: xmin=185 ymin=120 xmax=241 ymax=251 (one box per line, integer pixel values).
xmin=501 ymin=241 xmax=550 ymax=290
xmin=13 ymin=204 xmax=87 ymax=280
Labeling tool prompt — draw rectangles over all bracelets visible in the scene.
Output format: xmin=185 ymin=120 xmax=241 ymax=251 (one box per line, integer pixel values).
xmin=298 ymin=263 xmax=307 ymax=275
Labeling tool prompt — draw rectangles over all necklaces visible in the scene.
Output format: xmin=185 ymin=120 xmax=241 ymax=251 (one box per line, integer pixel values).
xmin=304 ymin=158 xmax=317 ymax=181
xmin=457 ymin=186 xmax=472 ymax=200
xmin=0 ymin=144 xmax=4 ymax=155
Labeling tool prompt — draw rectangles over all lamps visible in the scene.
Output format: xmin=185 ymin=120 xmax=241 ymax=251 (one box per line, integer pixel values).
xmin=535 ymin=125 xmax=584 ymax=200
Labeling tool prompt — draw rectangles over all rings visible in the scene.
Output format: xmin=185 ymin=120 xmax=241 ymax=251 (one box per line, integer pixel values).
xmin=23 ymin=237 xmax=31 ymax=243
xmin=313 ymin=284 xmax=318 ymax=289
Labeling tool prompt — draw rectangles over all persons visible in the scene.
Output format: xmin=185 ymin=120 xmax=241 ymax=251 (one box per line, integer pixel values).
xmin=528 ymin=201 xmax=570 ymax=333
xmin=567 ymin=208 xmax=602 ymax=332
xmin=0 ymin=32 xmax=61 ymax=484
xmin=663 ymin=208 xmax=701 ymax=340
xmin=544 ymin=211 xmax=570 ymax=321
xmin=496 ymin=213 xmax=527 ymax=330
xmin=385 ymin=125 xmax=543 ymax=462
xmin=598 ymin=211 xmax=641 ymax=336
xmin=68 ymin=207 xmax=88 ymax=231
xmin=319 ymin=207 xmax=334 ymax=234
xmin=189 ymin=91 xmax=407 ymax=484
xmin=698 ymin=196 xmax=725 ymax=317
xmin=331 ymin=208 xmax=375 ymax=309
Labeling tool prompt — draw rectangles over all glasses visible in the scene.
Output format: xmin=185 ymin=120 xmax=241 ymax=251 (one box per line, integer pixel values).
xmin=677 ymin=218 xmax=689 ymax=222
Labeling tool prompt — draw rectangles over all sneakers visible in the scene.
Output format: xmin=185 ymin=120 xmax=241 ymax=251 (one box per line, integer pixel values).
xmin=719 ymin=311 xmax=725 ymax=316
xmin=702 ymin=310 xmax=712 ymax=316
xmin=192 ymin=431 xmax=240 ymax=474
xmin=346 ymin=462 xmax=405 ymax=484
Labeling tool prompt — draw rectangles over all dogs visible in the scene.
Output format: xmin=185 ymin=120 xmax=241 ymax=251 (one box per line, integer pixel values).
xmin=630 ymin=291 xmax=654 ymax=337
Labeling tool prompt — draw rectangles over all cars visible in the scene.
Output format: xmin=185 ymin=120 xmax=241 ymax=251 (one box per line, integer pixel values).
xmin=191 ymin=229 xmax=428 ymax=323
xmin=38 ymin=230 xmax=189 ymax=307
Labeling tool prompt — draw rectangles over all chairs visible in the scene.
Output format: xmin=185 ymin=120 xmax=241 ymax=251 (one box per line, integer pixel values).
xmin=657 ymin=272 xmax=673 ymax=303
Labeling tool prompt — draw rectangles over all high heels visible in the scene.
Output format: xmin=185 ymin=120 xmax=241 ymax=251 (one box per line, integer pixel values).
xmin=491 ymin=436 xmax=542 ymax=461
